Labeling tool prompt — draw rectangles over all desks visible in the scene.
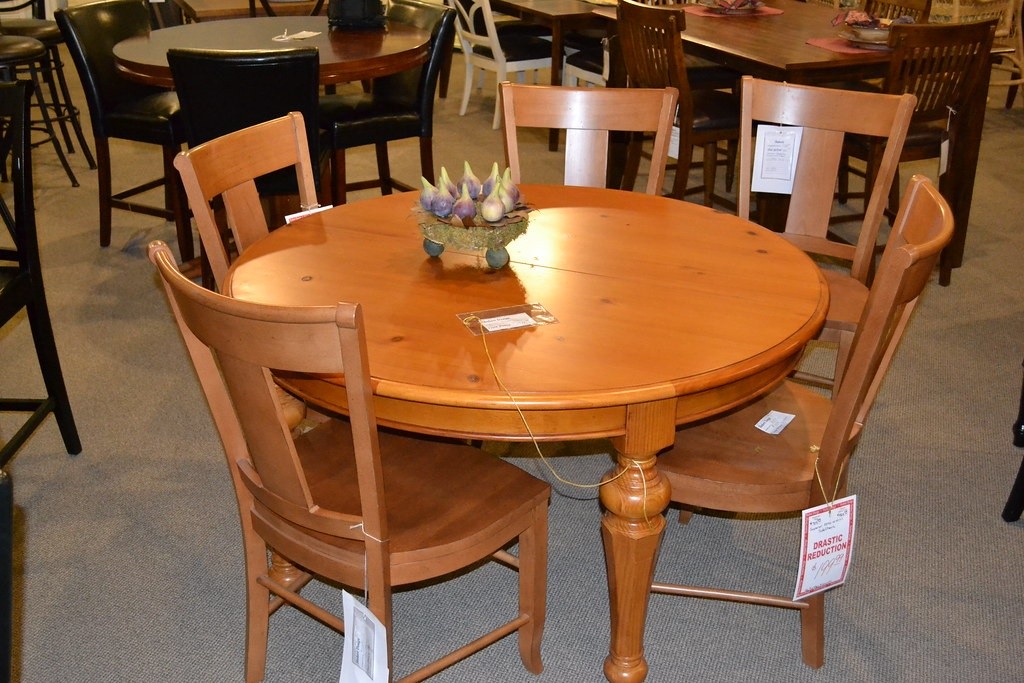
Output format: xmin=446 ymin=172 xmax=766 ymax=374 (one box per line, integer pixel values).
xmin=490 ymin=0 xmax=616 ymax=152
xmin=592 ymin=0 xmax=1016 ymax=232
xmin=112 ymin=16 xmax=432 ymax=86
xmin=320 ymin=0 xmax=456 ymax=208
xmin=220 ymin=183 xmax=832 ymax=683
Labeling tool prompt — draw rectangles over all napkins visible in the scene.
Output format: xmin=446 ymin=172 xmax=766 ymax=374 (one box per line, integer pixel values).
xmin=682 ymin=5 xmax=785 ymax=18
xmin=806 ymin=36 xmax=920 ymax=55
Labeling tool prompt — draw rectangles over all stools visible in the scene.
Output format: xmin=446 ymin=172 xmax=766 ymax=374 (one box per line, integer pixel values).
xmin=0 ymin=18 xmax=97 ymax=170
xmin=0 ymin=36 xmax=80 ymax=188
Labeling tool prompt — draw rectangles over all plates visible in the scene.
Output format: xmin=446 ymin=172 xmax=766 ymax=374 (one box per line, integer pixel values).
xmin=698 ymin=0 xmax=766 ymax=14
xmin=837 ymin=31 xmax=890 ymax=50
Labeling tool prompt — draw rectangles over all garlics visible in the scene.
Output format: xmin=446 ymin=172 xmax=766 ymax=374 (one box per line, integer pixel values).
xmin=420 ymin=161 xmax=519 ymax=222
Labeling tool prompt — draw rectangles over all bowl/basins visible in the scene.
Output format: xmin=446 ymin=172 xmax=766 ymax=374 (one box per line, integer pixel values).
xmin=846 ymin=25 xmax=889 ymax=41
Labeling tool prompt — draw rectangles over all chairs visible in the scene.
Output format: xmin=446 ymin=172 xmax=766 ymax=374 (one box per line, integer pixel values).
xmin=0 ymin=79 xmax=83 ymax=470
xmin=173 ymin=111 xmax=320 ymax=295
xmin=498 ymin=80 xmax=680 ymax=196
xmin=166 ymin=47 xmax=333 ymax=291
xmin=147 ymin=239 xmax=551 ymax=683
xmin=565 ymin=35 xmax=626 ymax=87
xmin=651 ymin=173 xmax=954 ymax=669
xmin=823 ymin=17 xmax=999 ymax=286
xmin=738 ymin=75 xmax=917 ymax=401
xmin=448 ymin=0 xmax=568 ymax=130
xmin=54 ymin=0 xmax=195 ymax=263
xmin=616 ymin=0 xmax=741 ymax=208
